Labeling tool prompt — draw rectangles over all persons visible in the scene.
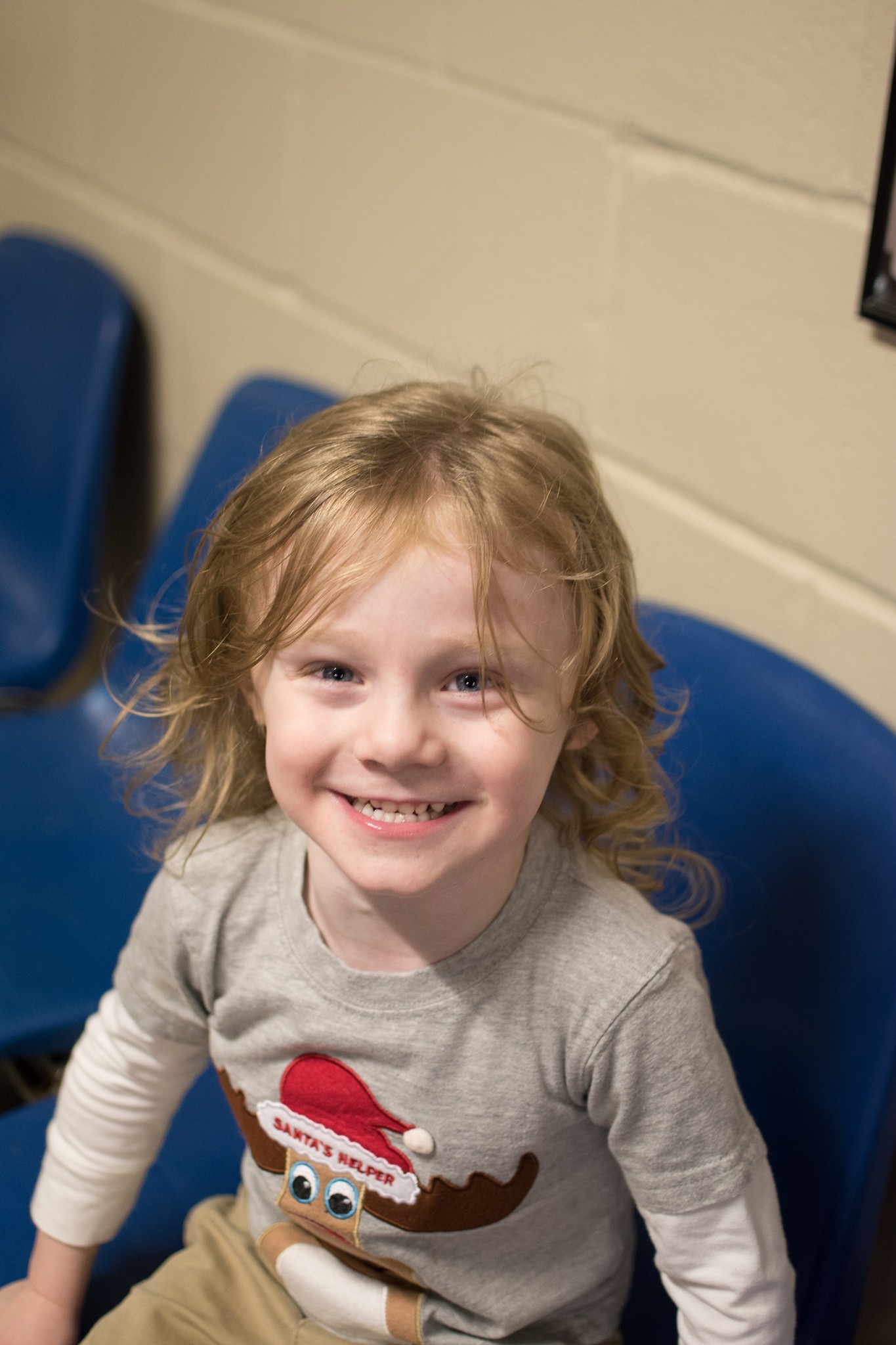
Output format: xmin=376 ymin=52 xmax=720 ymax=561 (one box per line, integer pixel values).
xmin=0 ymin=380 xmax=797 ymax=1345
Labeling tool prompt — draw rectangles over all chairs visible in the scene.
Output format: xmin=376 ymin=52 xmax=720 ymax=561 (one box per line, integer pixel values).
xmin=0 ymin=599 xmax=896 ymax=1345
xmin=0 ymin=231 xmax=128 ymax=687
xmin=0 ymin=377 xmax=342 ymax=1034
